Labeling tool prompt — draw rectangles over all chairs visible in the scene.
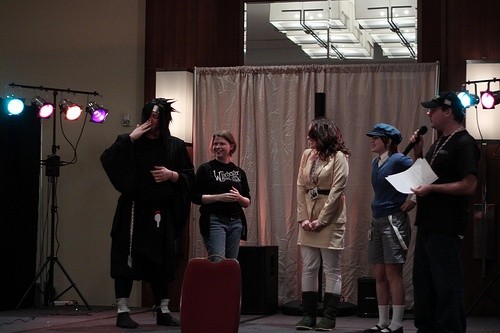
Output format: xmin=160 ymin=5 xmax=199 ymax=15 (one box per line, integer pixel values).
xmin=178 ymin=255 xmax=241 ymax=333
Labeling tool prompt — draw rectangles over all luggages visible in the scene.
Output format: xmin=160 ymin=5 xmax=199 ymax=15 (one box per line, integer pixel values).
xmin=179 ymin=253 xmax=242 ymax=333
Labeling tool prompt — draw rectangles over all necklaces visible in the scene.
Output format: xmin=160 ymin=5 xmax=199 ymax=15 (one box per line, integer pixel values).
xmin=431 ymin=125 xmax=463 ymax=165
xmin=312 ymin=158 xmax=320 ymax=177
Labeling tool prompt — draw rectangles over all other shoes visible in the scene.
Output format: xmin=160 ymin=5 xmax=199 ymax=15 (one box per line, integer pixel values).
xmin=363 ymin=324 xmax=404 ymax=333
xmin=116 ymin=312 xmax=141 ymax=329
xmin=156 ymin=310 xmax=179 ymax=326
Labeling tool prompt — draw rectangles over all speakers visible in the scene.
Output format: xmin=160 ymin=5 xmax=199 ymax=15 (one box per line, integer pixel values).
xmin=237 ymin=245 xmax=279 ymax=315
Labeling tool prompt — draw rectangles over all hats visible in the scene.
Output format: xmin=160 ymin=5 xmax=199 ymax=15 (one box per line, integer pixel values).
xmin=365 ymin=123 xmax=402 ymax=145
xmin=420 ymin=92 xmax=466 ymax=119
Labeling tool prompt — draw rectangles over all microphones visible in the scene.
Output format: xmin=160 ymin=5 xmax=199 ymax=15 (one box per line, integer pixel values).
xmin=402 ymin=126 xmax=428 ymax=156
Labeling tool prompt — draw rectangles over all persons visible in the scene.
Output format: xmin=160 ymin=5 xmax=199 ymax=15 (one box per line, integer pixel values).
xmin=365 ymin=91 xmax=482 ymax=333
xmin=193 ymin=130 xmax=251 ymax=263
xmin=100 ymin=98 xmax=194 ymax=328
xmin=297 ymin=118 xmax=349 ymax=332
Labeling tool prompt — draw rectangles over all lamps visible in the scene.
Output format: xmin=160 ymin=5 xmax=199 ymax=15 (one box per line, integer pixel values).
xmin=355 ymin=0 xmax=417 ymax=60
xmin=31 ymin=96 xmax=53 ymax=119
xmin=481 ymin=90 xmax=500 ymax=109
xmin=59 ymin=98 xmax=82 ymax=121
xmin=457 ymin=91 xmax=480 ymax=108
xmin=270 ymin=0 xmax=375 ymax=60
xmin=85 ymin=101 xmax=108 ymax=124
xmin=4 ymin=96 xmax=25 ymax=117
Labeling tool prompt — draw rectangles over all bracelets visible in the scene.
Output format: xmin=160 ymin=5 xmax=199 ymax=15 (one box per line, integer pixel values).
xmin=170 ymin=170 xmax=174 ymax=180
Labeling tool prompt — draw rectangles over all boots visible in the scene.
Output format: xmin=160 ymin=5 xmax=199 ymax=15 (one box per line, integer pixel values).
xmin=314 ymin=292 xmax=341 ymax=331
xmin=296 ymin=291 xmax=319 ymax=329
